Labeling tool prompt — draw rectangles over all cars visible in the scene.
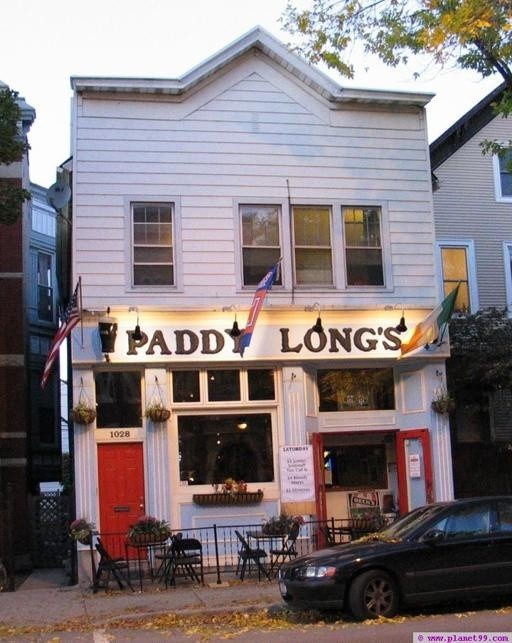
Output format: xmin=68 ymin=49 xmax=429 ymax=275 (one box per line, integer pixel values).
xmin=278 ymin=495 xmax=512 ymax=621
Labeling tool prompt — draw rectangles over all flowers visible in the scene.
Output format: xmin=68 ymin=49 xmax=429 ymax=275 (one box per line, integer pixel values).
xmin=68 ymin=517 xmax=95 ymax=540
xmin=258 ymin=511 xmax=305 ymax=529
xmin=125 ymin=515 xmax=171 ymax=537
xmin=351 ymin=510 xmax=380 ymax=523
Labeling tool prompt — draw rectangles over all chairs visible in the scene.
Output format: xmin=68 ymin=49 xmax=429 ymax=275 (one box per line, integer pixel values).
xmin=94 ymin=524 xmax=300 ymax=594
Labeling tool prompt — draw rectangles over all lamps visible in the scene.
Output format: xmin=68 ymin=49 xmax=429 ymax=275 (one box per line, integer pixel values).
xmin=128 ymin=305 xmax=143 ymax=341
xmin=304 ymin=302 xmax=324 ymax=333
xmin=222 ymin=303 xmax=241 ymax=337
xmin=384 ymin=302 xmax=407 ymax=333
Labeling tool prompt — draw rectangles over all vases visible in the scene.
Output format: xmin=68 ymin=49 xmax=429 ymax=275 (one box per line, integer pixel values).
xmin=78 ymin=533 xmax=92 ymax=545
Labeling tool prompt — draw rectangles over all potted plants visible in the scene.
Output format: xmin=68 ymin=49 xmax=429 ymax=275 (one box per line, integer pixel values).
xmin=147 ymin=404 xmax=169 ymax=422
xmin=430 ymin=396 xmax=455 ymax=414
xmin=68 ymin=403 xmax=96 ymax=425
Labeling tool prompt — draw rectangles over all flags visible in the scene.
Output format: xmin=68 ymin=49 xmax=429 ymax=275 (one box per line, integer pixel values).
xmin=39 ymin=280 xmax=81 ymax=391
xmin=397 ymin=286 xmax=459 ymax=361
xmin=240 ymin=263 xmax=279 ymax=357
